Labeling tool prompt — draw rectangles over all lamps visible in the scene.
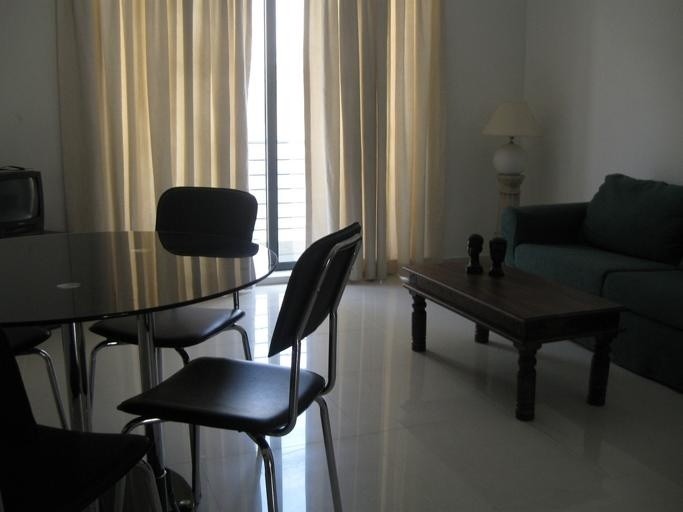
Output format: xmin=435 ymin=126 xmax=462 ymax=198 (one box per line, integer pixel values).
xmin=482 ymin=100 xmax=546 ymax=236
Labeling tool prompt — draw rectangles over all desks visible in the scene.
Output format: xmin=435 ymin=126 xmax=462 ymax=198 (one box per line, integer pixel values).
xmin=399 ymin=255 xmax=627 ymax=422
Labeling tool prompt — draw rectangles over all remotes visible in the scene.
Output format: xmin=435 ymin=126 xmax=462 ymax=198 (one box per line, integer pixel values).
xmin=7 ymin=166 xmax=26 ymax=170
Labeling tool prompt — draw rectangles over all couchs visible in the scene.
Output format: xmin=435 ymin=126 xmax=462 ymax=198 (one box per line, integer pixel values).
xmin=504 ymin=174 xmax=683 ymax=397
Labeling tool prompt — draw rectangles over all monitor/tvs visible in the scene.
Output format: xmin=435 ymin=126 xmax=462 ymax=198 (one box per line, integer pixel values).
xmin=0 ymin=169 xmax=44 ymax=239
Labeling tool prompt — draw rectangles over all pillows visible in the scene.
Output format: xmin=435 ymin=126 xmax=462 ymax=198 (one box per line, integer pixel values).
xmin=585 ymin=174 xmax=683 ymax=263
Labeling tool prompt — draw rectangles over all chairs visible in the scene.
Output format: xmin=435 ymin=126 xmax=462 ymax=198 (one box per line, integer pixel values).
xmin=1 ymin=231 xmax=277 ymax=512
xmin=122 ymin=222 xmax=366 ymax=512
xmin=1 ymin=324 xmax=152 ymax=512
xmin=6 ymin=324 xmax=73 ymax=430
xmin=89 ymin=186 xmax=258 ymax=497
